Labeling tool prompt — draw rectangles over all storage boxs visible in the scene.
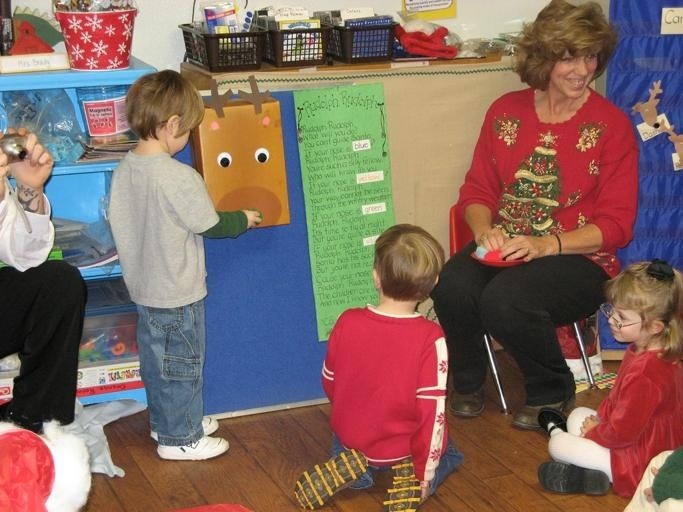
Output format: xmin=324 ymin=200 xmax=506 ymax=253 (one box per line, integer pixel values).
xmin=1 ymin=368 xmax=20 ymax=405
xmin=75 ymin=359 xmax=142 ymax=395
xmin=77 ymin=313 xmax=140 ymax=363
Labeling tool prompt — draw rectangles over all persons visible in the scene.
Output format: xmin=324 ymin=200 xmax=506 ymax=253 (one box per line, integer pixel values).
xmin=622 ymin=445 xmax=683 ymax=512
xmin=108 ymin=69 xmax=261 ymax=462
xmin=293 ymin=223 xmax=463 ymax=512
xmin=540 ymin=260 xmax=683 ymax=495
xmin=0 ymin=126 xmax=89 ymax=437
xmin=426 ymin=0 xmax=640 ymax=432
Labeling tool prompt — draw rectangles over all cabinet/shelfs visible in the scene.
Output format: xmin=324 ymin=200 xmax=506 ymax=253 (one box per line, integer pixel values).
xmin=178 ymin=58 xmax=598 ymax=356
xmin=0 ymin=50 xmax=158 ymax=413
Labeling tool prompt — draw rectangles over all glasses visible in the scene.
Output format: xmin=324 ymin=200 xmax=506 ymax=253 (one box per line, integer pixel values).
xmin=598 ymin=302 xmax=644 ymax=331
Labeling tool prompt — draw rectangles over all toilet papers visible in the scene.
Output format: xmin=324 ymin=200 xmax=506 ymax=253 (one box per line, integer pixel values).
xmin=213 ymin=2 xmax=238 ymax=26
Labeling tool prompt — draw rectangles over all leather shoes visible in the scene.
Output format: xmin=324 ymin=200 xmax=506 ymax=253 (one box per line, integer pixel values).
xmin=510 ymin=390 xmax=577 ymax=433
xmin=536 ymin=460 xmax=610 ymax=497
xmin=537 ymin=406 xmax=568 ymax=436
xmin=448 ymin=382 xmax=486 ymax=418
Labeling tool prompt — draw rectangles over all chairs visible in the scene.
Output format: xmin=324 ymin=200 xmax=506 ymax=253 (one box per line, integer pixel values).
xmin=447 ymin=205 xmax=598 ymax=421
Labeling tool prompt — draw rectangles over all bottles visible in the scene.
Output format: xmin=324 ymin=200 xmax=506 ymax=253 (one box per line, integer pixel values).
xmin=73 ymin=86 xmax=132 ymax=144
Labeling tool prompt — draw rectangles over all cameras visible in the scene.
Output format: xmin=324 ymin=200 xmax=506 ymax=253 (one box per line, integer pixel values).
xmin=0 ymin=135 xmax=29 ymax=164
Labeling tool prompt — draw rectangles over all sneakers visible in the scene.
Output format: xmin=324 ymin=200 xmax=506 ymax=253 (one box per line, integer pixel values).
xmin=155 ymin=434 xmax=231 ymax=463
xmin=292 ymin=447 xmax=370 ymax=511
xmin=382 ymin=456 xmax=423 ymax=512
xmin=149 ymin=415 xmax=220 ymax=443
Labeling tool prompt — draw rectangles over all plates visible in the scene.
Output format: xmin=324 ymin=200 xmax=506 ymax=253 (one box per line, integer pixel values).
xmin=469 ymin=251 xmax=522 ymax=267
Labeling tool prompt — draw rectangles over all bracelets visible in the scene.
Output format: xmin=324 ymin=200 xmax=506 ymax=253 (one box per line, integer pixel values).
xmin=552 ymin=230 xmax=563 ymax=255
